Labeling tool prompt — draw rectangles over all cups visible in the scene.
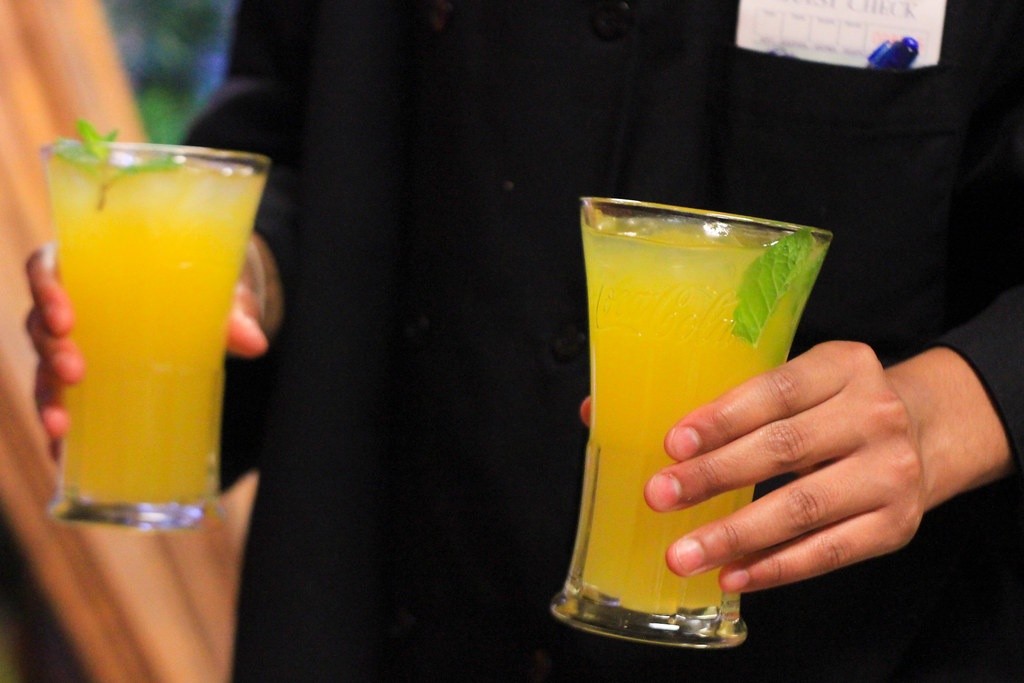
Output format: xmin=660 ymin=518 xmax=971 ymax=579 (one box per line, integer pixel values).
xmin=34 ymin=137 xmax=273 ymax=539
xmin=550 ymin=193 xmax=834 ymax=652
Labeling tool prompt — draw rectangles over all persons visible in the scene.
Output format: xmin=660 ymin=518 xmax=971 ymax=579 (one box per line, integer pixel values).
xmin=24 ymin=0 xmax=1024 ymax=683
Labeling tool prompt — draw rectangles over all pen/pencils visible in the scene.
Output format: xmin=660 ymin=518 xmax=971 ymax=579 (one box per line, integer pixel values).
xmin=867 ymin=36 xmax=919 ymax=70
